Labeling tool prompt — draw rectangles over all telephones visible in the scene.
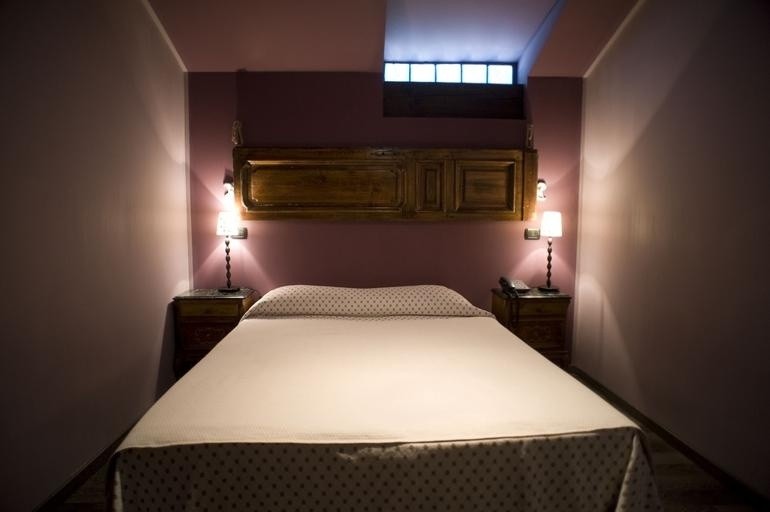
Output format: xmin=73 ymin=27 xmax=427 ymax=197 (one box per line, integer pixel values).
xmin=499 ymin=276 xmax=531 ymax=293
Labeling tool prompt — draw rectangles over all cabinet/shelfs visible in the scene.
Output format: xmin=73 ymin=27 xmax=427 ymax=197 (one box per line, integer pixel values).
xmin=229 ymin=141 xmax=541 ymax=225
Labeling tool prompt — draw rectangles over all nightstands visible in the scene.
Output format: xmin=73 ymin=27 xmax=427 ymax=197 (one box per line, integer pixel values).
xmin=492 ymin=283 xmax=575 ymax=373
xmin=171 ymin=287 xmax=259 ymax=381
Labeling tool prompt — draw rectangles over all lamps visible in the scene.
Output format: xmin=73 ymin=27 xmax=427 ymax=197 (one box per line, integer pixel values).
xmin=210 ymin=210 xmax=257 ymax=292
xmin=538 ymin=208 xmax=564 ymax=295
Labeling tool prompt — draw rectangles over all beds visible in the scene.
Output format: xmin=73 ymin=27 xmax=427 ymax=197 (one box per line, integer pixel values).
xmin=99 ymin=283 xmax=677 ymax=512
